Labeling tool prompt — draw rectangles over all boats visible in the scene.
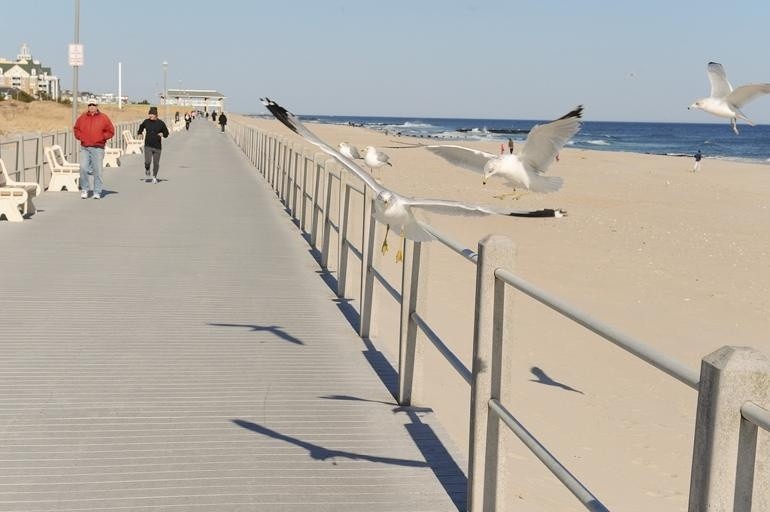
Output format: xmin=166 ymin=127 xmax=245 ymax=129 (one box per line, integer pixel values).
xmin=454 ymin=126 xmax=530 ymax=133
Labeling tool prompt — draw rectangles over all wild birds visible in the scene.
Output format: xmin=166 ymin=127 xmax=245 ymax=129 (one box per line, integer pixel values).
xmin=259 ymin=95 xmax=571 ymax=265
xmin=376 ymin=105 xmax=586 ymax=204
xmin=336 ymin=140 xmax=363 ymax=164
xmin=362 ymin=145 xmax=395 ymax=179
xmin=683 ymin=59 xmax=769 ymax=137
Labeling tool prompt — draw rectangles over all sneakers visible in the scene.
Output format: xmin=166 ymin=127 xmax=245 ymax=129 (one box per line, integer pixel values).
xmin=92 ymin=194 xmax=100 ymax=199
xmin=81 ymin=190 xmax=88 ymax=199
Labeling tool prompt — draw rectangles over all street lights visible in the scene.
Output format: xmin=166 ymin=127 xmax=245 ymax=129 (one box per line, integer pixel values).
xmin=162 ymin=58 xmax=170 ymax=111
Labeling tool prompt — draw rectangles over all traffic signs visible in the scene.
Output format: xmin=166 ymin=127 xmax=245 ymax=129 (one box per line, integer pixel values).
xmin=177 ymin=77 xmax=191 ymax=111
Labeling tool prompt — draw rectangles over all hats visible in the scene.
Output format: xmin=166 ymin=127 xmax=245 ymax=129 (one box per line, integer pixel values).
xmin=88 ymin=98 xmax=98 ymax=106
xmin=149 ymin=107 xmax=157 ymax=115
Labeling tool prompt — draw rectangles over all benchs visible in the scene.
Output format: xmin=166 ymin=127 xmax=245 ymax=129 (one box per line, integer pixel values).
xmin=0 ymin=158 xmax=41 ymax=223
xmin=103 ymin=143 xmax=124 ymax=168
xmin=122 ymin=130 xmax=144 ymax=155
xmin=44 ymin=144 xmax=86 ymax=194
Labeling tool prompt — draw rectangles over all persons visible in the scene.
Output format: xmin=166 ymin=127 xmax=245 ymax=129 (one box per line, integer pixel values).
xmin=175 ymin=108 xmax=227 ymax=132
xmin=507 ymin=138 xmax=514 ymax=154
xmin=692 ymin=149 xmax=703 ymax=173
xmin=137 ymin=106 xmax=169 ymax=184
xmin=74 ymin=99 xmax=114 ymax=199
